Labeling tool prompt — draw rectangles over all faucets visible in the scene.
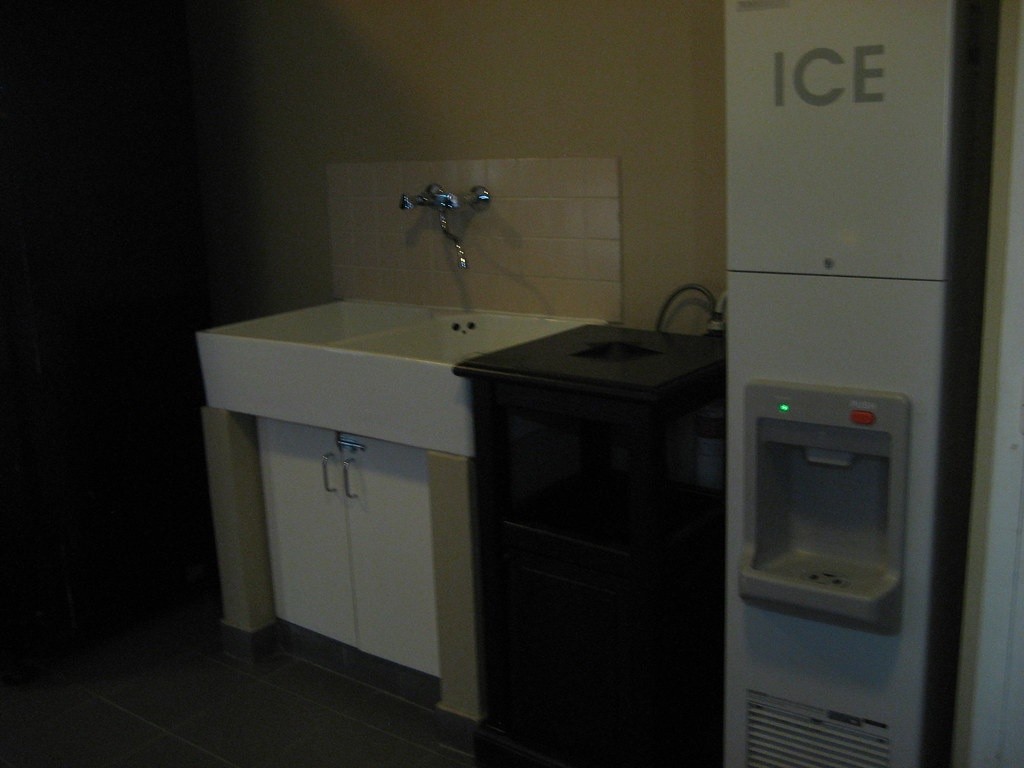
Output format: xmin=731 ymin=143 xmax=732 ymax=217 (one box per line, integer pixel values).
xmin=398 ymin=191 xmax=470 ymax=271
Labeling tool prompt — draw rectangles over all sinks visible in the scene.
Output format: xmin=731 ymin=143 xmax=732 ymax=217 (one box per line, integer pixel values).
xmin=194 ymin=299 xmax=608 ymax=462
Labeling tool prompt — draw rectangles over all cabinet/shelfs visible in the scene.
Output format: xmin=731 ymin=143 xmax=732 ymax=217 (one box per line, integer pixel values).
xmin=255 ymin=418 xmax=440 ymax=678
